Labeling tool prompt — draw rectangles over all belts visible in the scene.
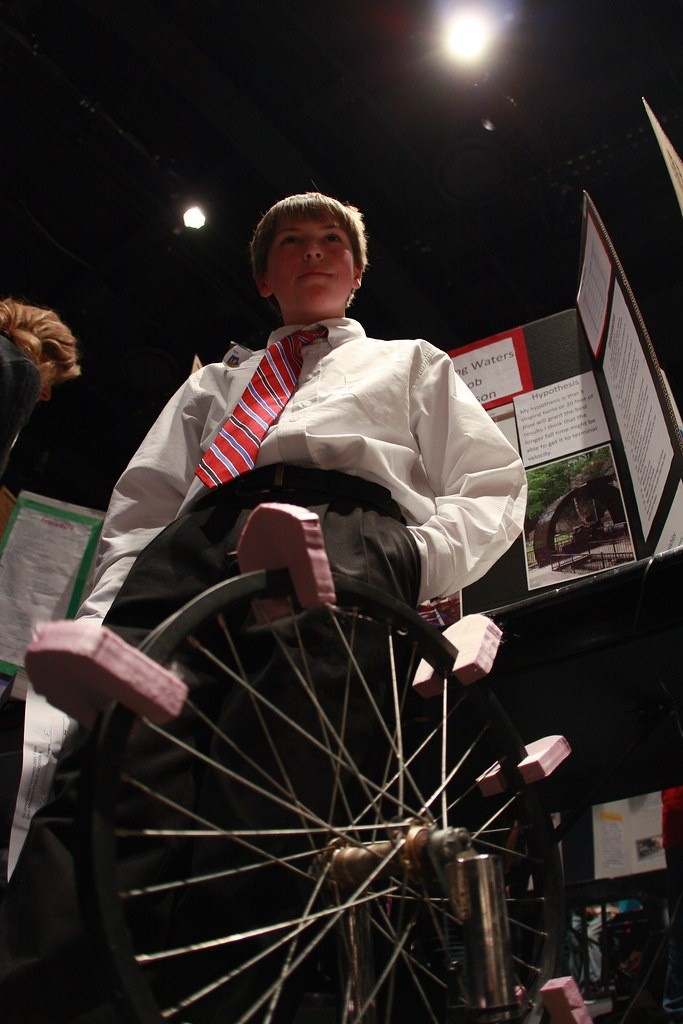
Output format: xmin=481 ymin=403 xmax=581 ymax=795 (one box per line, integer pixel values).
xmin=194 ymin=463 xmax=391 ymax=504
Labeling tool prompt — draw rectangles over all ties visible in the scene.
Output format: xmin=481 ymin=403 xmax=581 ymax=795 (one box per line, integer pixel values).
xmin=196 ymin=328 xmax=328 ymax=489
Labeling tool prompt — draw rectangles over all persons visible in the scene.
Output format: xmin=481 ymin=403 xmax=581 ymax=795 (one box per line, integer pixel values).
xmin=0 ymin=192 xmax=526 ymax=1024
xmin=566 ymin=785 xmax=683 ymax=1024
xmin=0 ymin=295 xmax=83 ymax=487
